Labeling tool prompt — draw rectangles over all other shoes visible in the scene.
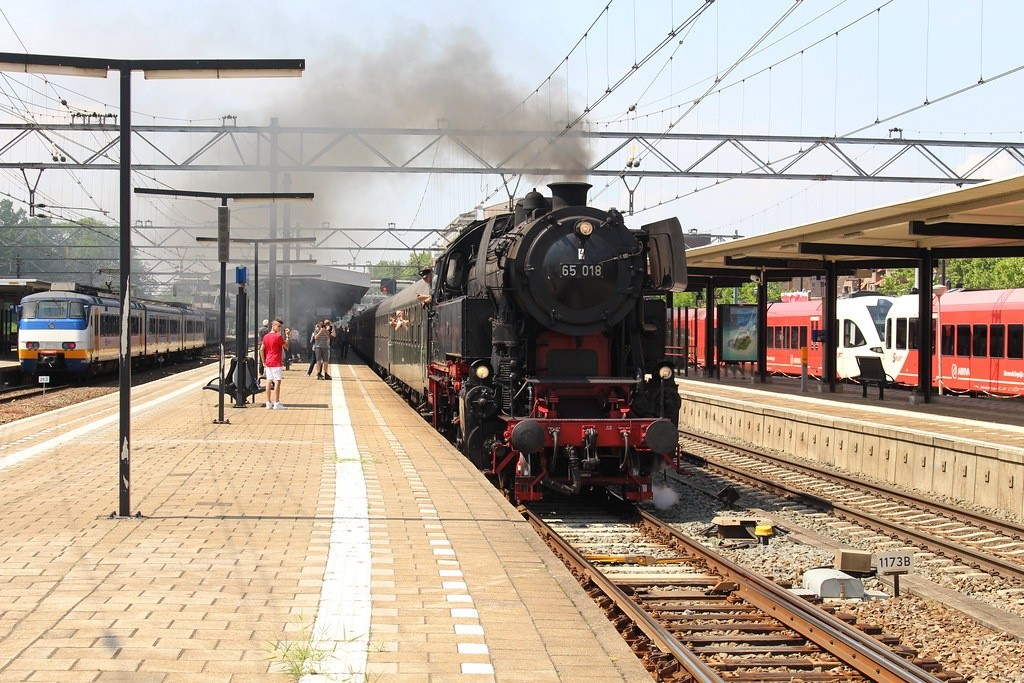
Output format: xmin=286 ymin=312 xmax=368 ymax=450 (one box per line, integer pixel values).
xmin=317 ymin=373 xmax=324 ymax=380
xmin=324 ymin=374 xmax=332 ymax=380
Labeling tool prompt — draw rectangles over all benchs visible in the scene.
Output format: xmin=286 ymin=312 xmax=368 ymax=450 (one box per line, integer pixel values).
xmin=203 ymin=358 xmax=275 ymax=403
xmin=855 ymin=356 xmax=905 ymax=399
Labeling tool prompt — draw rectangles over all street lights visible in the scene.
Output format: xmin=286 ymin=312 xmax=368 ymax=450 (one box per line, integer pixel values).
xmin=934 ymin=283 xmax=947 ymax=396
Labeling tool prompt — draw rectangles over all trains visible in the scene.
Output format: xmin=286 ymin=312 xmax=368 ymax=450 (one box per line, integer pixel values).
xmin=338 ymin=181 xmax=689 ymax=511
xmin=14 ymin=290 xmax=207 ymax=387
xmin=665 ymin=286 xmax=1024 ymax=402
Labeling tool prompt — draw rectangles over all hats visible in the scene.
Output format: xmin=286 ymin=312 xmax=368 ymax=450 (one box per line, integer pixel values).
xmin=263 ymin=319 xmax=269 ymax=327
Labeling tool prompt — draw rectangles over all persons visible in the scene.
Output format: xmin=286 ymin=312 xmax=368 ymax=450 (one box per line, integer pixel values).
xmin=260 ymin=319 xmax=290 ymax=409
xmin=289 ymin=327 xmax=302 ymax=362
xmin=258 ymin=319 xmax=271 ymax=376
xmin=306 ymin=320 xmax=351 ymax=380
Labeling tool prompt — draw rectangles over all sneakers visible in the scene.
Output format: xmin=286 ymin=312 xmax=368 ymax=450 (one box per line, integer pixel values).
xmin=266 ymin=401 xmax=272 ymax=410
xmin=273 ymin=402 xmax=288 ymax=411
